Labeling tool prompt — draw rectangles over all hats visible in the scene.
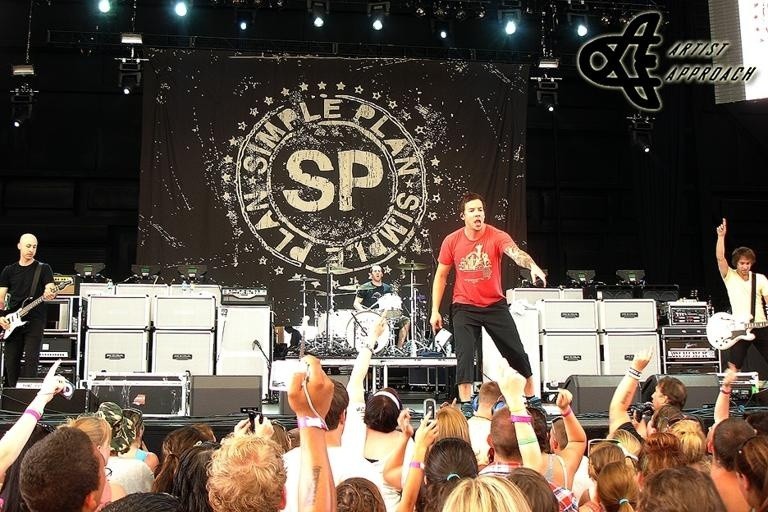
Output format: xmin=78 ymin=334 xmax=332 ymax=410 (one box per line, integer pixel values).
xmin=95 ymin=401 xmax=137 ymax=451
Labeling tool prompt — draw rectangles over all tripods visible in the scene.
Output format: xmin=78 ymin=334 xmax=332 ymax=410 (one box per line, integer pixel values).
xmin=288 ymin=247 xmax=441 ymax=357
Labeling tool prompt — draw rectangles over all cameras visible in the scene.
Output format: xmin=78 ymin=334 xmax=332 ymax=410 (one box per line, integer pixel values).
xmin=56 ymin=374 xmax=75 ymax=400
xmin=297 ymin=359 xmax=311 ymax=383
xmin=632 ymin=402 xmax=655 ymax=425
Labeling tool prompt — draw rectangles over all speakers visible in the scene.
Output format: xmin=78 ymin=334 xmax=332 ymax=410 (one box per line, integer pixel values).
xmin=562 ymin=375 xmax=639 ymax=412
xmin=280 ymin=376 xmax=350 ymax=415
xmin=641 ymin=375 xmax=720 ymax=408
xmin=190 ymin=375 xmax=262 ymax=417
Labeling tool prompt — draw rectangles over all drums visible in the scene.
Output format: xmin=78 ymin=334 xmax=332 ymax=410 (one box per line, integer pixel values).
xmin=429 ymin=328 xmax=453 ymax=352
xmin=379 ymin=293 xmax=401 ymax=317
xmin=345 ymin=311 xmax=390 ymax=354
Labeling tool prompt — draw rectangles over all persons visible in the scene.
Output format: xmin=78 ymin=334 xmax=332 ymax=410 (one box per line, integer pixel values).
xmin=430 ymin=193 xmax=548 ymax=420
xmin=353 ymin=264 xmax=410 ymax=356
xmin=715 ymin=217 xmax=768 ymax=373
xmin=0 ymin=233 xmax=56 ymax=388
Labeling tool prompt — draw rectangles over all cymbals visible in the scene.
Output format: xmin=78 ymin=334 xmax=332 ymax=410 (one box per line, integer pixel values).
xmin=403 ymin=284 xmax=424 ymax=286
xmin=313 ymin=266 xmax=349 ymax=275
xmin=338 ymin=284 xmax=376 ymax=290
xmin=395 ymin=263 xmax=430 ymax=270
xmin=288 ymin=278 xmax=319 ymax=281
xmin=300 ymin=289 xmax=330 ymax=297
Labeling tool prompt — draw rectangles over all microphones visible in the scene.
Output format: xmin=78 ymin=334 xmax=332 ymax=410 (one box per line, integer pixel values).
xmin=422 ymin=236 xmax=427 ymax=252
xmin=536 ymin=276 xmax=543 ymax=287
xmin=346 ymin=237 xmax=359 ymax=247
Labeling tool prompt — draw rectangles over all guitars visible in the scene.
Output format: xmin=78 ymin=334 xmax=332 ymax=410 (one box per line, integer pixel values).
xmin=0 ymin=280 xmax=72 ymax=339
xmin=705 ymin=312 xmax=768 ymax=350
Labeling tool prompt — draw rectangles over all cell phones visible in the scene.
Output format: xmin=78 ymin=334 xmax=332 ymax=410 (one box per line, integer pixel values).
xmin=424 ymin=398 xmax=436 ymax=429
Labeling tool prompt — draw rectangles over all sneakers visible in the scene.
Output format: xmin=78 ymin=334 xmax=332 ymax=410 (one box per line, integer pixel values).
xmin=460 ymin=402 xmax=474 ymax=422
xmin=528 ymin=395 xmax=553 ymax=424
